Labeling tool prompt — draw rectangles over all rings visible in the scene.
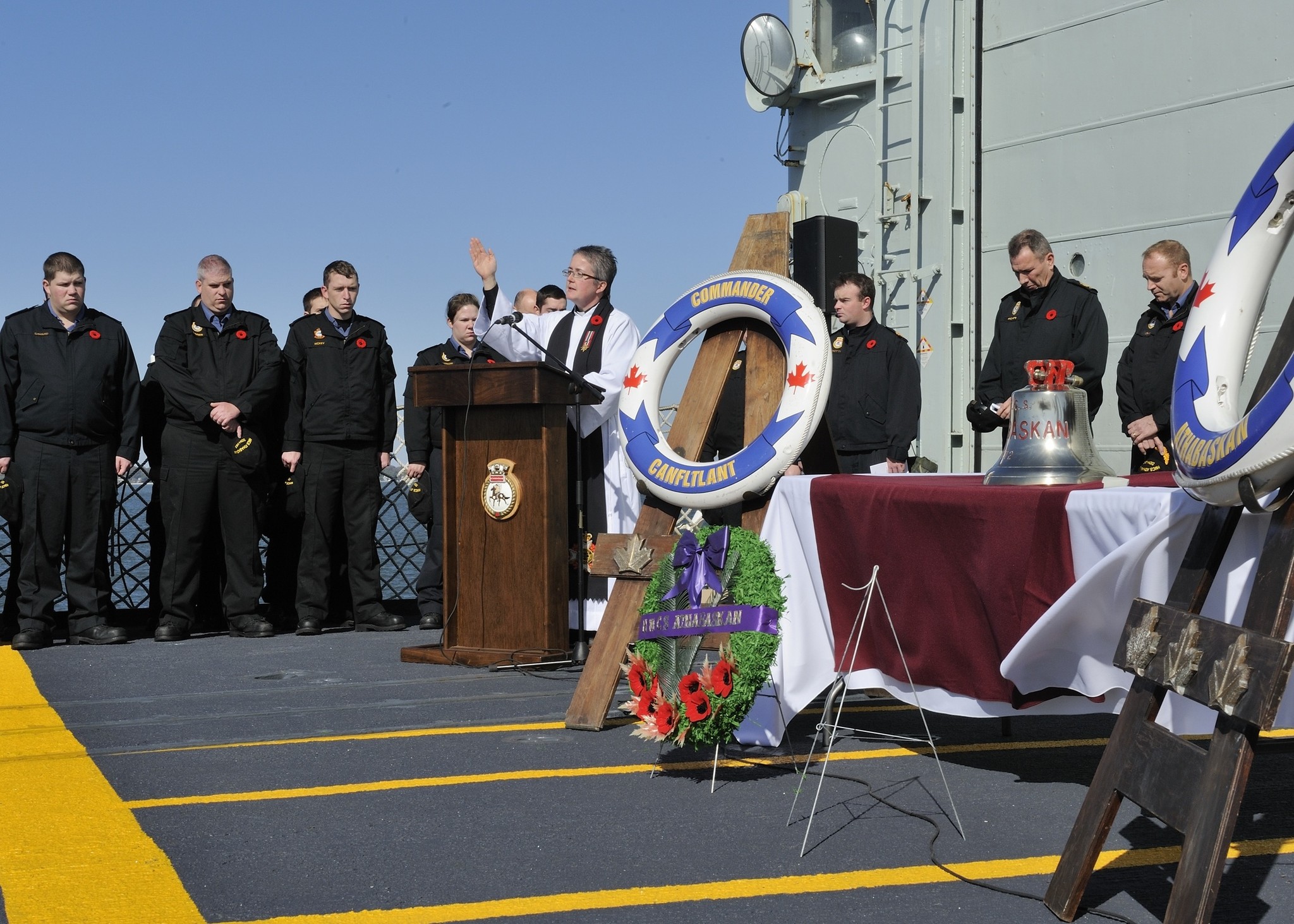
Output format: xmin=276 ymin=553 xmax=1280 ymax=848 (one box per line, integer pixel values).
xmin=1133 ymin=432 xmax=1137 ymax=438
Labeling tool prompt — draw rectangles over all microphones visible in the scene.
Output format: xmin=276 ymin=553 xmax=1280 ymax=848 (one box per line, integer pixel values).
xmin=495 ymin=312 xmax=524 ymax=325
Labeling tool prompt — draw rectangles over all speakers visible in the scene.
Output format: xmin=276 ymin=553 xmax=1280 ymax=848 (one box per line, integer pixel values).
xmin=792 ymin=214 xmax=858 ymax=314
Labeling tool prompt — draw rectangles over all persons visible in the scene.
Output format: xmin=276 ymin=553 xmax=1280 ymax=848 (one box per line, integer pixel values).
xmin=469 ymin=235 xmax=642 ymax=651
xmin=403 ymin=293 xmax=510 ymax=629
xmin=0 ymin=250 xmax=142 ymax=647
xmin=1116 ymin=239 xmax=1200 ymax=475
xmin=141 ymin=254 xmax=281 ymax=642
xmin=978 ymin=229 xmax=1108 ymax=452
xmin=801 ymin=273 xmax=921 ymax=474
xmin=511 ymin=284 xmax=567 ymax=315
xmin=280 ymin=261 xmax=411 ymax=635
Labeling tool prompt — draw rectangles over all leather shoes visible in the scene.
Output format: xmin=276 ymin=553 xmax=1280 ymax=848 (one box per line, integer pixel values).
xmin=154 ymin=621 xmax=191 ymax=641
xmin=295 ymin=615 xmax=322 ymax=636
xmin=11 ymin=628 xmax=54 ymax=650
xmin=68 ymin=624 xmax=129 ymax=645
xmin=419 ymin=612 xmax=443 ymax=629
xmin=354 ymin=611 xmax=407 ymax=632
xmin=228 ymin=617 xmax=274 ymax=638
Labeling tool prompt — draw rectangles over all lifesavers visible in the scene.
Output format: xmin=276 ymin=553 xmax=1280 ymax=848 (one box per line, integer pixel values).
xmin=616 ymin=268 xmax=836 ymax=511
xmin=1171 ymin=123 xmax=1294 ymax=504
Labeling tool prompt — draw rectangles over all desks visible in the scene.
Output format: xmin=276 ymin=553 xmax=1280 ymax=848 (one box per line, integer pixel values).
xmin=735 ymin=468 xmax=1293 ymax=748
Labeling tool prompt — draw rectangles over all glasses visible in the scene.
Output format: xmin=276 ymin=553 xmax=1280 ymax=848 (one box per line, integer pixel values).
xmin=562 ymin=269 xmax=607 ymax=282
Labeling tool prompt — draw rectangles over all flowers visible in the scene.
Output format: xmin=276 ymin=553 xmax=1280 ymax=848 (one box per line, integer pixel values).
xmin=619 ymin=644 xmax=734 ymax=746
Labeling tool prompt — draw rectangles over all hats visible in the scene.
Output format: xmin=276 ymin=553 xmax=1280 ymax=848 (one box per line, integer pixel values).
xmin=1131 ymin=444 xmax=1170 ymax=475
xmin=276 ymin=462 xmax=305 ymax=521
xmin=219 ymin=426 xmax=267 ymax=474
xmin=966 ymin=399 xmax=1003 ymax=433
xmin=407 ymin=469 xmax=432 ymax=524
xmin=0 ymin=465 xmax=26 ymax=524
